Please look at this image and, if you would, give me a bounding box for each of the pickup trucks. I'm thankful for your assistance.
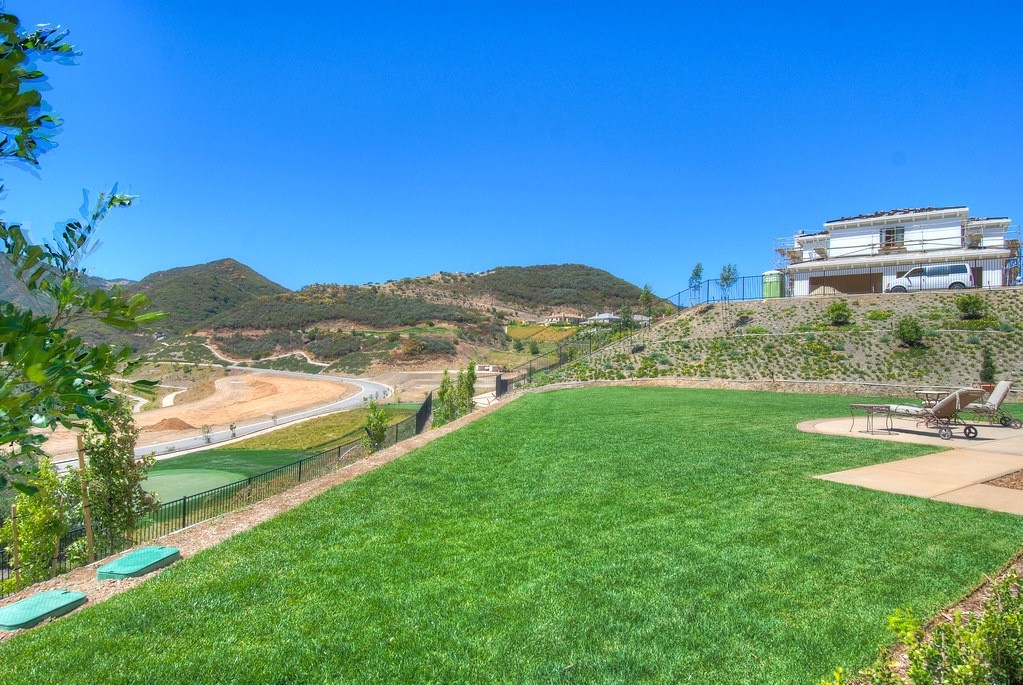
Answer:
[884,262,977,291]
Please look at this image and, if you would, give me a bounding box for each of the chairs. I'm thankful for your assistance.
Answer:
[885,389,985,437]
[923,381,1022,427]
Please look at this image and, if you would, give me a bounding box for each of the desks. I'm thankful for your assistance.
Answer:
[849,404,893,436]
[915,391,950,429]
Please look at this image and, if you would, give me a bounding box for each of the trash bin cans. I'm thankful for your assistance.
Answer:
[761,269,783,299]
